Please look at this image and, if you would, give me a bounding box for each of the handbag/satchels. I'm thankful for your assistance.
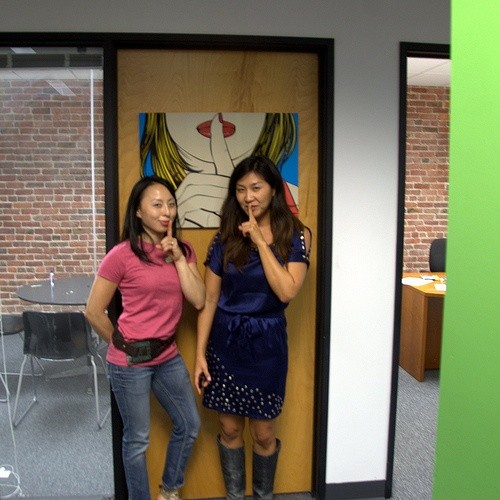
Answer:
[111,324,176,366]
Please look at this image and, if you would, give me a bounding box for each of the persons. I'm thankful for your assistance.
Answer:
[84,176,207,500]
[193,156,313,500]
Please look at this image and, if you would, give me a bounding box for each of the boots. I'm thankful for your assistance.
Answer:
[252,438,281,500]
[216,434,246,500]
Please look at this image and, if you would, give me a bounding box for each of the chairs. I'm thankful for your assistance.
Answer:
[0,314,45,402]
[12,311,111,430]
[430,237,447,271]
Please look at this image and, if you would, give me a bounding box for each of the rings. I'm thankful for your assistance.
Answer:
[171,241,174,246]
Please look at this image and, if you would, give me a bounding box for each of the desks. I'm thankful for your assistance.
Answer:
[17,274,107,392]
[402,271,447,381]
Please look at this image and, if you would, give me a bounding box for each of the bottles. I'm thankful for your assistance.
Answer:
[49,270,55,286]
